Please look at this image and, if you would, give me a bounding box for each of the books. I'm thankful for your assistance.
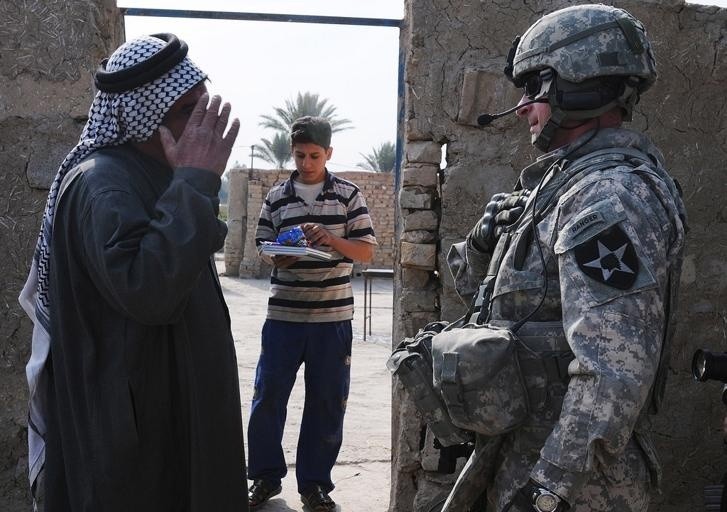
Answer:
[262,245,332,262]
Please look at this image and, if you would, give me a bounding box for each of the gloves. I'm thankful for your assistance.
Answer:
[472,190,531,251]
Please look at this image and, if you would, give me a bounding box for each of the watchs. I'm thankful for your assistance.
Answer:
[521,481,570,512]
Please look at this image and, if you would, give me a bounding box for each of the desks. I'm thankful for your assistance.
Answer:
[361,268,395,342]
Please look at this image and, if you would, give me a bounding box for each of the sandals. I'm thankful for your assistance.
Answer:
[246,478,283,508]
[299,484,335,511]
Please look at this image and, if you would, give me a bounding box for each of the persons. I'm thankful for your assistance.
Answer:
[17,34,249,512]
[247,116,379,512]
[385,3,689,512]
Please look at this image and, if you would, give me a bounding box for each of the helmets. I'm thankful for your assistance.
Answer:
[507,2,658,89]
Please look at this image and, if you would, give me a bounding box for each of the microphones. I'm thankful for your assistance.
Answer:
[477,93,548,126]
